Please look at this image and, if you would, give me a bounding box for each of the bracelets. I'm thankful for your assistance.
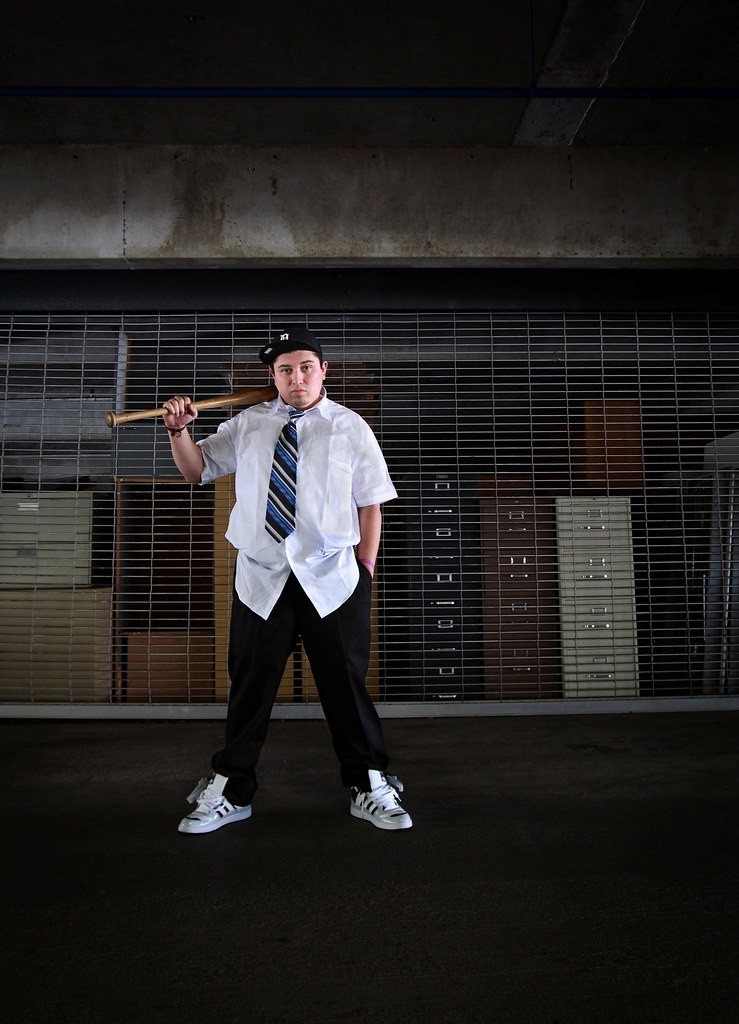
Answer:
[359,559,375,568]
[166,424,187,437]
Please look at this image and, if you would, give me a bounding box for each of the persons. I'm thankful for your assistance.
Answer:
[162,329,413,833]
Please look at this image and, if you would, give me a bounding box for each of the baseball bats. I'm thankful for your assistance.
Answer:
[105,385,278,428]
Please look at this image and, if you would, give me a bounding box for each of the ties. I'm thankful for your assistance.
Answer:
[264,409,306,544]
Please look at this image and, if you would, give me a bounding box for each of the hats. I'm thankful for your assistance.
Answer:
[259,329,323,364]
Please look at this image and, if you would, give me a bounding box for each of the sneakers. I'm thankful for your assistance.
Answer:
[178,773,252,834]
[349,767,412,829]
